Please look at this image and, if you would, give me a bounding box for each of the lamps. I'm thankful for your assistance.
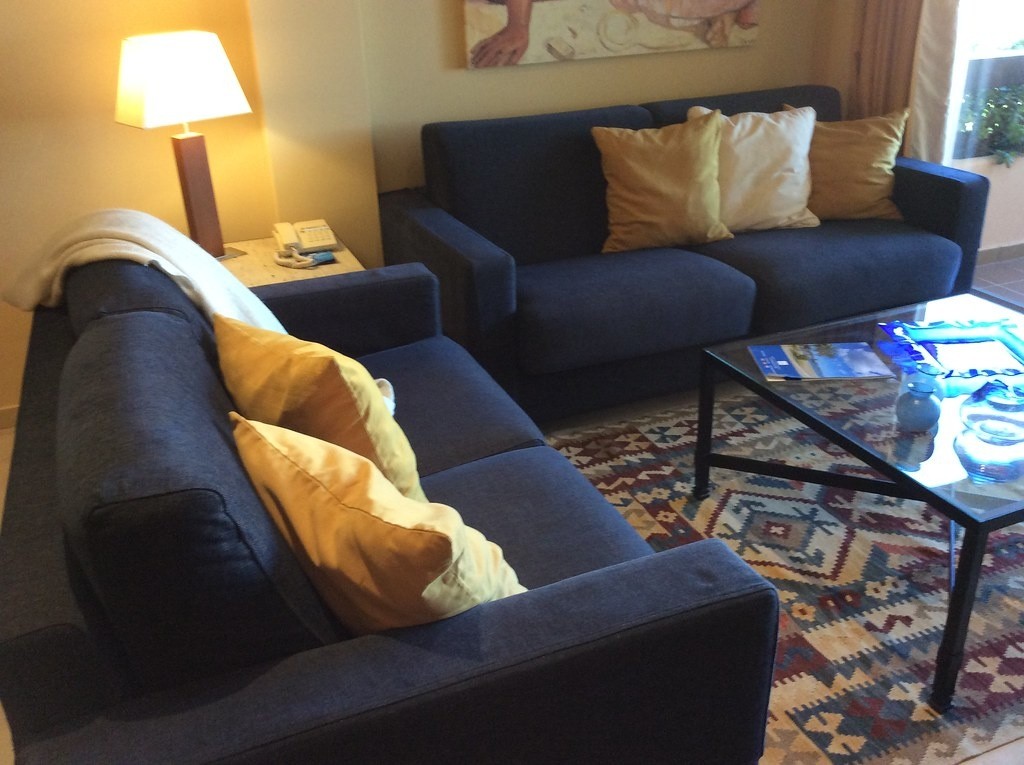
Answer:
[114,31,253,258]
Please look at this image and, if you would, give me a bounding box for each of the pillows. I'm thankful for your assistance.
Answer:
[222,414,529,637]
[210,313,430,518]
[672,96,821,235]
[780,102,912,226]
[591,106,736,255]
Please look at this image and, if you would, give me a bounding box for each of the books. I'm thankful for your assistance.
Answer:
[747,342,897,382]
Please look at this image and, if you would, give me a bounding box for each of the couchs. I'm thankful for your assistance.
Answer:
[1,261,780,765]
[378,85,991,424]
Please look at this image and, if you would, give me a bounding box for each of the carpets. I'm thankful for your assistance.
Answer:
[542,377,1023,763]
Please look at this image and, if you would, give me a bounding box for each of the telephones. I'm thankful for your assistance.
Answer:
[271,219,336,256]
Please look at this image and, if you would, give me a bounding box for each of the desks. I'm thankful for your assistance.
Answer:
[695,292,1024,715]
[216,231,366,287]
